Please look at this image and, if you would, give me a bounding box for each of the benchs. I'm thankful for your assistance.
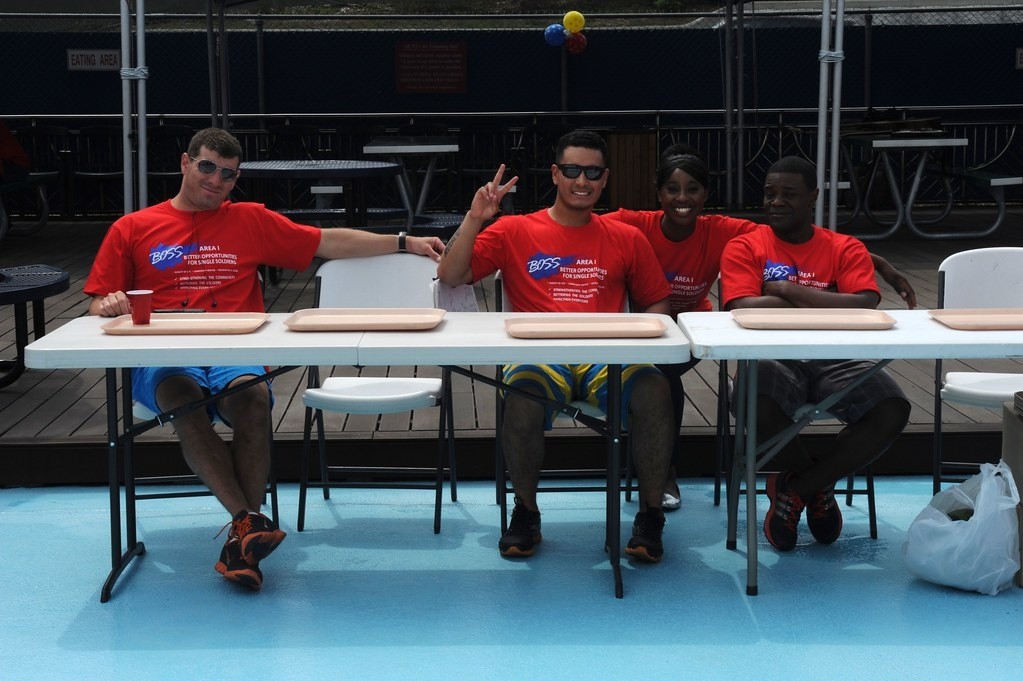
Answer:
[348,205,469,246]
[0,265,69,388]
[926,165,1023,239]
[824,176,850,227]
[0,171,59,241]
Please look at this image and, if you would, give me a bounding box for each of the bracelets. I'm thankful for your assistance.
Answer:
[398,231,409,253]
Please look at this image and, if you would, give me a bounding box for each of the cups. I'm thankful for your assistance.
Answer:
[126,290,153,325]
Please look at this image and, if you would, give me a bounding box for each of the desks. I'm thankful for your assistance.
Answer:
[23,312,691,604]
[237,134,459,286]
[839,135,968,240]
[677,308,1023,596]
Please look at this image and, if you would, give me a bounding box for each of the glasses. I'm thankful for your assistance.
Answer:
[190,156,237,182]
[556,164,605,180]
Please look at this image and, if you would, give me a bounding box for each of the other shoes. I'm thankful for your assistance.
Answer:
[662,484,681,508]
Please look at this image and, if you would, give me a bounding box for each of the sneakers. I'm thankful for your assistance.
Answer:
[499,496,542,556]
[213,512,287,567]
[764,473,805,551]
[806,479,842,543]
[214,537,263,590]
[625,512,666,561]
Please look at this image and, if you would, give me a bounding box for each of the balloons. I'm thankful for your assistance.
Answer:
[563,11,585,33]
[544,24,566,47]
[565,33,587,55]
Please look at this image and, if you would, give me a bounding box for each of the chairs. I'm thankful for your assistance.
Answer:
[494,267,879,550]
[933,247,1023,495]
[134,252,459,536]
[15,125,614,220]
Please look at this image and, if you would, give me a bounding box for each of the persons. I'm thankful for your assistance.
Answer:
[436,132,676,564]
[718,156,912,551]
[320,381,323,386]
[81,127,444,592]
[598,144,919,509]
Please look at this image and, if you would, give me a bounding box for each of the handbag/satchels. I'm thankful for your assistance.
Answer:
[904,458,1021,595]
